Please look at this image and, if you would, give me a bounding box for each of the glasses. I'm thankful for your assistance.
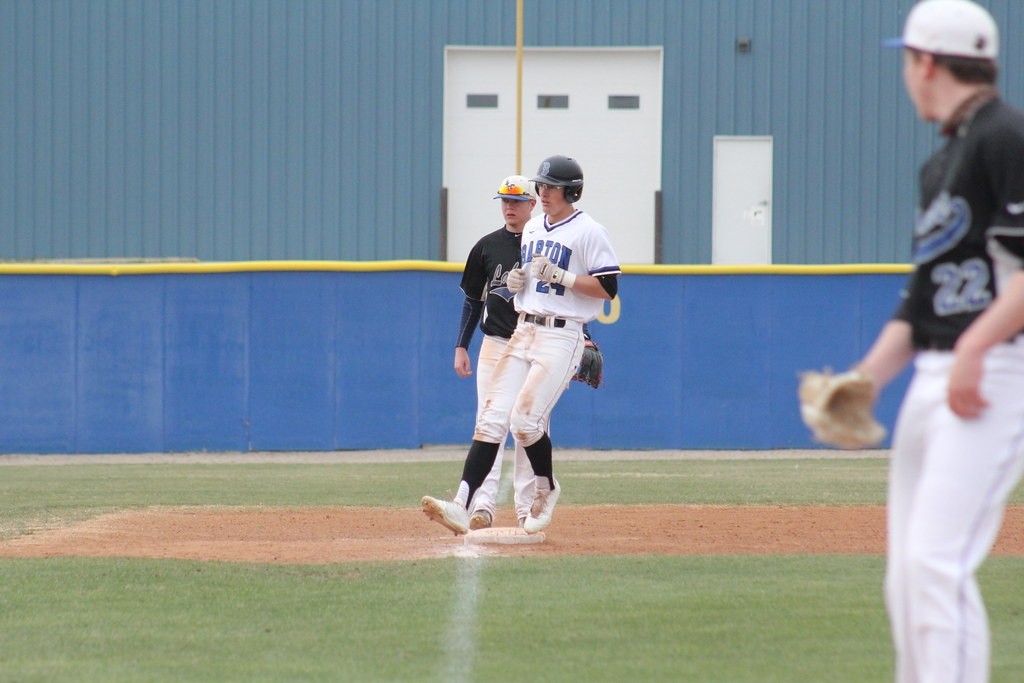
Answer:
[499,185,530,195]
[537,182,565,190]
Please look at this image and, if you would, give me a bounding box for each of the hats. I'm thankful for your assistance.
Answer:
[882,1,999,57]
[494,174,537,202]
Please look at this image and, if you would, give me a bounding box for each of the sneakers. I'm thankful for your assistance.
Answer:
[523,476,561,533]
[422,495,471,534]
[519,517,527,527]
[469,512,491,530]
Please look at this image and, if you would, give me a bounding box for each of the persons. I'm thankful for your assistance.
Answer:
[797,1,1024,683]
[421,155,622,535]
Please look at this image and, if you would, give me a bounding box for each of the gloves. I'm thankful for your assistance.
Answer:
[532,253,576,289]
[507,268,527,292]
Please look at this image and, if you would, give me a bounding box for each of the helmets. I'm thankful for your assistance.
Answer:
[527,155,583,202]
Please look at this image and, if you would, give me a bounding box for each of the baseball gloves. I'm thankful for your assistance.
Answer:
[797,370,890,453]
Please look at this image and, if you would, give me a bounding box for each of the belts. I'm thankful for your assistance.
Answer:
[524,313,566,328]
